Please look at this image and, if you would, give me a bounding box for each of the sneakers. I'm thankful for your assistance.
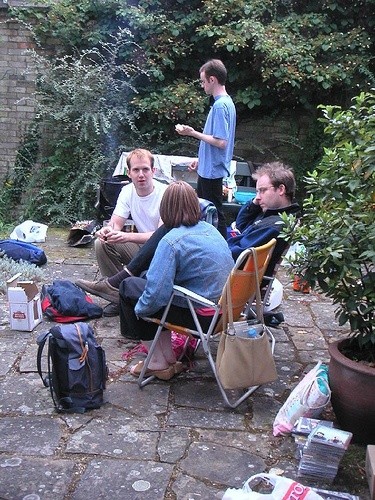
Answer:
[75,277,122,305]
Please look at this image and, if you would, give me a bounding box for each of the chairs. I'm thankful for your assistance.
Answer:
[94,180,300,409]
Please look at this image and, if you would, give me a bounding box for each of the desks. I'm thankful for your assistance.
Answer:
[153,153,252,188]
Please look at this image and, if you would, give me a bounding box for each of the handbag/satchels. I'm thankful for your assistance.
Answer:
[273,361,331,436]
[10,220,49,243]
[67,220,99,247]
[0,240,47,265]
[41,279,102,323]
[222,472,324,500]
[215,247,279,390]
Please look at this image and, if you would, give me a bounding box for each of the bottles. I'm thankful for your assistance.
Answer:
[226,318,266,338]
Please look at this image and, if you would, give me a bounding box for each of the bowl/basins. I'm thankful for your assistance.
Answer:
[234,192,257,205]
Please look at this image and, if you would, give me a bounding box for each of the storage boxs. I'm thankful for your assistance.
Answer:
[67,220,99,247]
[7,273,43,332]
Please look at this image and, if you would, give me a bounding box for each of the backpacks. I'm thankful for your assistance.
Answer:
[36,322,109,413]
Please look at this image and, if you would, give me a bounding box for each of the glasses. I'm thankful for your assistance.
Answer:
[256,186,275,194]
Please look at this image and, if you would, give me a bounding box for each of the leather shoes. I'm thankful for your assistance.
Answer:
[102,301,119,317]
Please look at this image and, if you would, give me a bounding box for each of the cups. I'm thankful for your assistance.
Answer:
[103,220,114,243]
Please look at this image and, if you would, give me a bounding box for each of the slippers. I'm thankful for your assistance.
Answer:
[129,362,182,380]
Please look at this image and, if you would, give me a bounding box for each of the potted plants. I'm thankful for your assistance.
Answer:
[274,93,375,445]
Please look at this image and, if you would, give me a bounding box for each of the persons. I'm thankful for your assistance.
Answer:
[117,181,238,381]
[95,149,171,318]
[175,59,237,242]
[76,162,300,306]
[313,431,345,445]
